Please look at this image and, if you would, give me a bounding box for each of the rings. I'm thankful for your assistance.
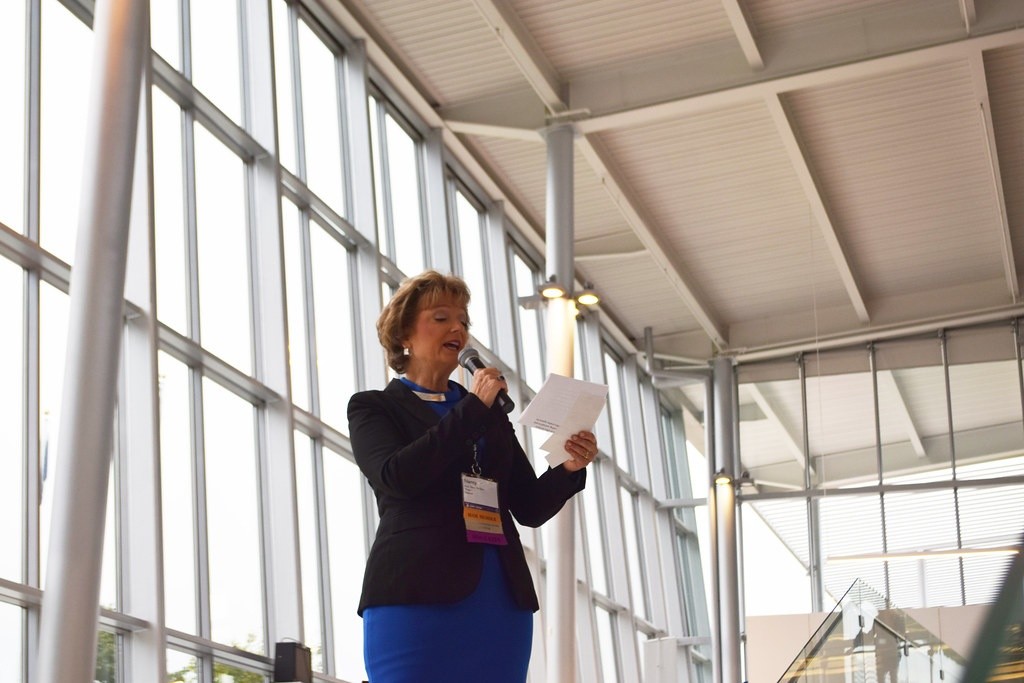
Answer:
[497,376,505,381]
[584,452,589,459]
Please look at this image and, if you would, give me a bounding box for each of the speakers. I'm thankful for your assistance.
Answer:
[274,642,312,683]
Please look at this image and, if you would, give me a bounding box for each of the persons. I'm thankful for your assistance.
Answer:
[346,269,598,683]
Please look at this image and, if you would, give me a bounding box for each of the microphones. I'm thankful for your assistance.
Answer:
[457,348,515,413]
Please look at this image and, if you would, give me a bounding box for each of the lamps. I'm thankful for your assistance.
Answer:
[536,281,568,299]
[574,288,602,306]
